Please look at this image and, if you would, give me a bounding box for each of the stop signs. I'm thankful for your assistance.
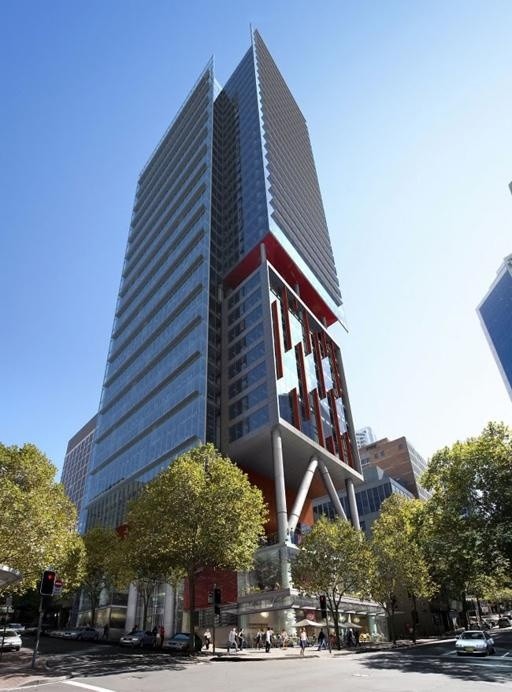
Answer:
[54,579,62,595]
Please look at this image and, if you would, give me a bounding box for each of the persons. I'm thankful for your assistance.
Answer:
[346,628,353,648]
[299,626,309,656]
[102,623,110,640]
[329,633,337,648]
[312,632,315,639]
[270,627,275,633]
[353,627,362,647]
[265,626,272,652]
[281,628,288,648]
[238,627,249,651]
[203,627,212,650]
[317,627,327,650]
[254,628,260,650]
[159,625,165,648]
[260,628,266,640]
[152,625,158,647]
[226,627,240,652]
[131,624,138,631]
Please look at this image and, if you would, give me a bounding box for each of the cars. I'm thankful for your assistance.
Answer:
[120,630,159,648]
[0,623,100,651]
[162,632,202,656]
[456,630,495,655]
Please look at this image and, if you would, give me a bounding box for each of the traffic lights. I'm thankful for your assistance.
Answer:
[215,588,221,604]
[40,570,56,597]
[320,595,327,610]
